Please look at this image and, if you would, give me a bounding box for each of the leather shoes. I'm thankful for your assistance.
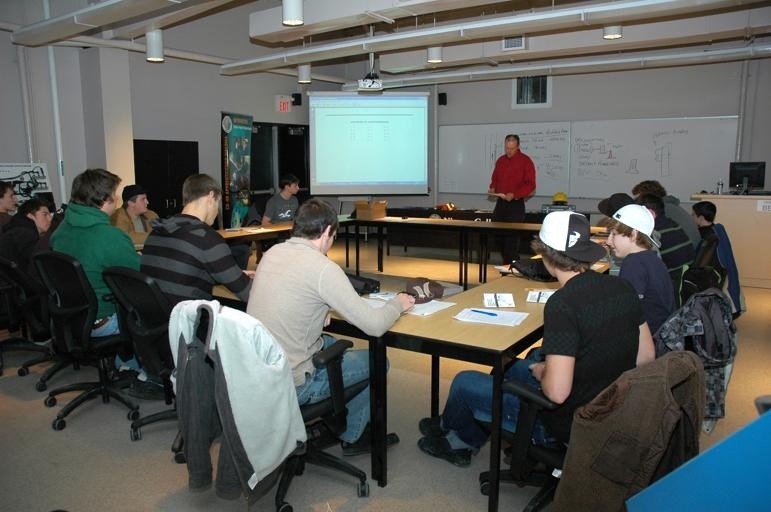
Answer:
[342,423,399,456]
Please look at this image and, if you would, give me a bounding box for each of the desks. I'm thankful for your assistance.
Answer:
[375,257,611,510]
[353,207,581,291]
[212,270,375,480]
[135,211,363,279]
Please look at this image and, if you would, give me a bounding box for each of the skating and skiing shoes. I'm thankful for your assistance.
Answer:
[420,416,446,436]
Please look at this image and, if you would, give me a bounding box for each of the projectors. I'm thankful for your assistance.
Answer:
[341,78,385,93]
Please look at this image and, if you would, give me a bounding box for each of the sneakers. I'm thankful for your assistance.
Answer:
[419,436,472,465]
[129,373,166,400]
[112,365,140,386]
[34,332,56,345]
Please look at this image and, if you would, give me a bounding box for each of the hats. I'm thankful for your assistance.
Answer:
[406,278,444,304]
[123,185,151,201]
[599,194,636,218]
[613,204,660,248]
[539,211,607,262]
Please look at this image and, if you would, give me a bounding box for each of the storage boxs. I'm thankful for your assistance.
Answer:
[354,198,390,226]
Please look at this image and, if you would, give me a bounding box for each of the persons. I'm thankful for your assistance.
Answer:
[631,191,698,313]
[0,181,18,230]
[48,167,169,401]
[416,208,658,467]
[594,192,636,221]
[525,204,678,363]
[139,173,251,317]
[260,173,301,228]
[0,197,64,346]
[110,182,162,247]
[689,201,721,277]
[631,177,704,262]
[486,132,538,223]
[245,195,417,458]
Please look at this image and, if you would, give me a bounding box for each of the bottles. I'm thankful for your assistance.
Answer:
[717,179,724,195]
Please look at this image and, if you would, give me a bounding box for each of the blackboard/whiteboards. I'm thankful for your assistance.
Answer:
[438,119,570,200]
[569,114,738,204]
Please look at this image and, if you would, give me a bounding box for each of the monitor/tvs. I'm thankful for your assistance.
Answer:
[728,160,766,196]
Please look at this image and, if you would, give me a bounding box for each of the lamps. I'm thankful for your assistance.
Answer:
[8,0,196,49]
[342,45,771,95]
[218,1,756,79]
[601,24,623,42]
[427,46,443,66]
[297,63,311,85]
[143,23,165,64]
[281,0,305,29]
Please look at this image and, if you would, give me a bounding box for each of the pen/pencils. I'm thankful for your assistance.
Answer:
[537,292,541,303]
[471,310,497,316]
[494,293,499,307]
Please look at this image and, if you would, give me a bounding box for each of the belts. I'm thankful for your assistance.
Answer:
[94,316,109,329]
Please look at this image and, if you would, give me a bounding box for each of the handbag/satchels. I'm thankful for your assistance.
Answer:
[346,273,380,293]
[510,254,559,281]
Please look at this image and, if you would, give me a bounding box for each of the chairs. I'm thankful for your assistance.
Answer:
[33,249,144,432]
[1,245,98,391]
[478,347,706,512]
[654,288,738,430]
[169,300,370,512]
[101,267,184,464]
[682,235,720,296]
